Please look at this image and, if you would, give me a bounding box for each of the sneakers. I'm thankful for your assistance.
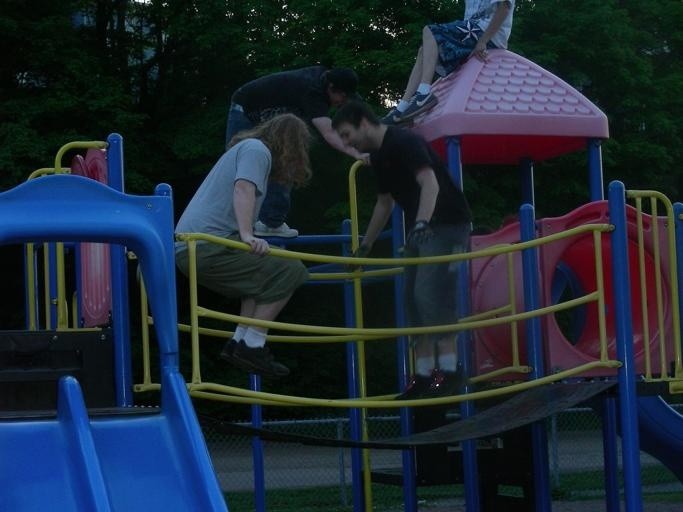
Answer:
[380,90,439,128]
[253,219,300,238]
[221,339,290,381]
[394,369,459,401]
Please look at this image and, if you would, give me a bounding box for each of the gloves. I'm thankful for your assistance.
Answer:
[407,219,437,249]
[343,246,370,282]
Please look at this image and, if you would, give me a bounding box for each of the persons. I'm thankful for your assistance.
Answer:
[223,64,372,241]
[172,111,313,380]
[376,0,515,124]
[330,99,476,400]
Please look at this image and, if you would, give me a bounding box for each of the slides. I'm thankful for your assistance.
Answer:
[0,370,228,512]
